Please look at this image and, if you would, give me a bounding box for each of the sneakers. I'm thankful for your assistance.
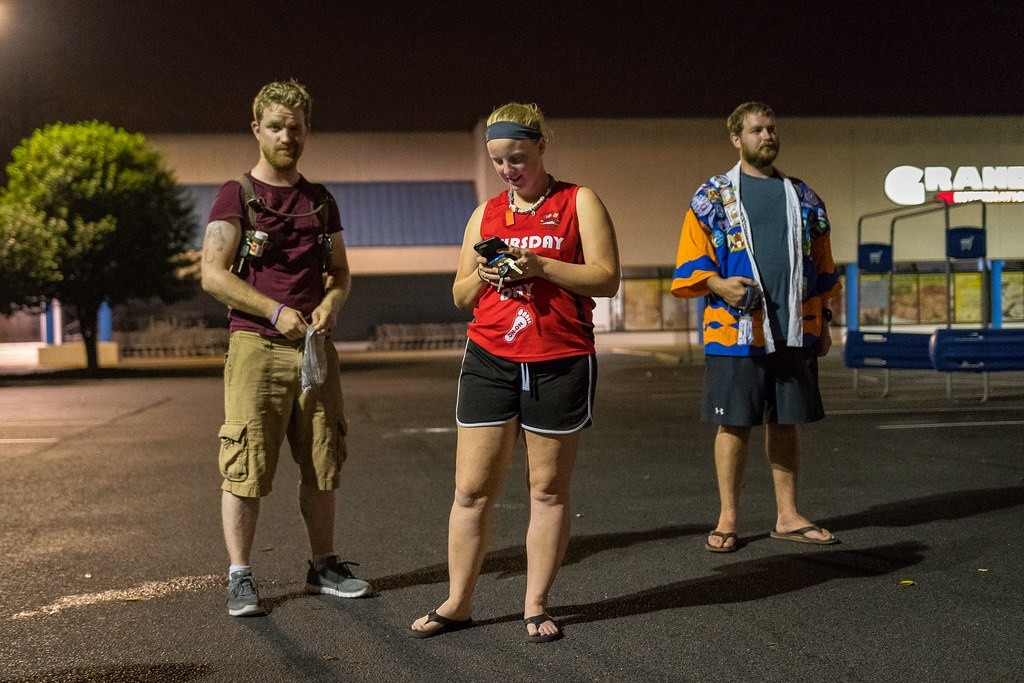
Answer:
[224,569,265,617]
[304,555,373,597]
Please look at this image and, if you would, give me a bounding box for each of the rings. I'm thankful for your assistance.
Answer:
[488,279,491,284]
[328,328,332,335]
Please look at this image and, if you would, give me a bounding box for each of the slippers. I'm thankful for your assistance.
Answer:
[704,530,737,552]
[406,609,472,639]
[770,525,839,544]
[523,614,557,643]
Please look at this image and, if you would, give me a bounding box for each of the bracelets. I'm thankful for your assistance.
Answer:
[477,268,486,282]
[271,304,285,325]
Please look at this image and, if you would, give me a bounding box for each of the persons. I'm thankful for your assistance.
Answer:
[671,102,840,552]
[408,102,620,641]
[201,79,371,617]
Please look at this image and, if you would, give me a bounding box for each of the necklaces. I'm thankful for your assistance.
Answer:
[508,174,555,213]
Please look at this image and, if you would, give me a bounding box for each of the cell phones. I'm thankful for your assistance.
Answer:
[473,236,508,262]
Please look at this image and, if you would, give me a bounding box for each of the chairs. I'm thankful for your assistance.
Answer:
[60,315,228,356]
[375,322,468,351]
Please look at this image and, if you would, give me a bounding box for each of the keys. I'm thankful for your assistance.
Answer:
[497,258,523,292]
